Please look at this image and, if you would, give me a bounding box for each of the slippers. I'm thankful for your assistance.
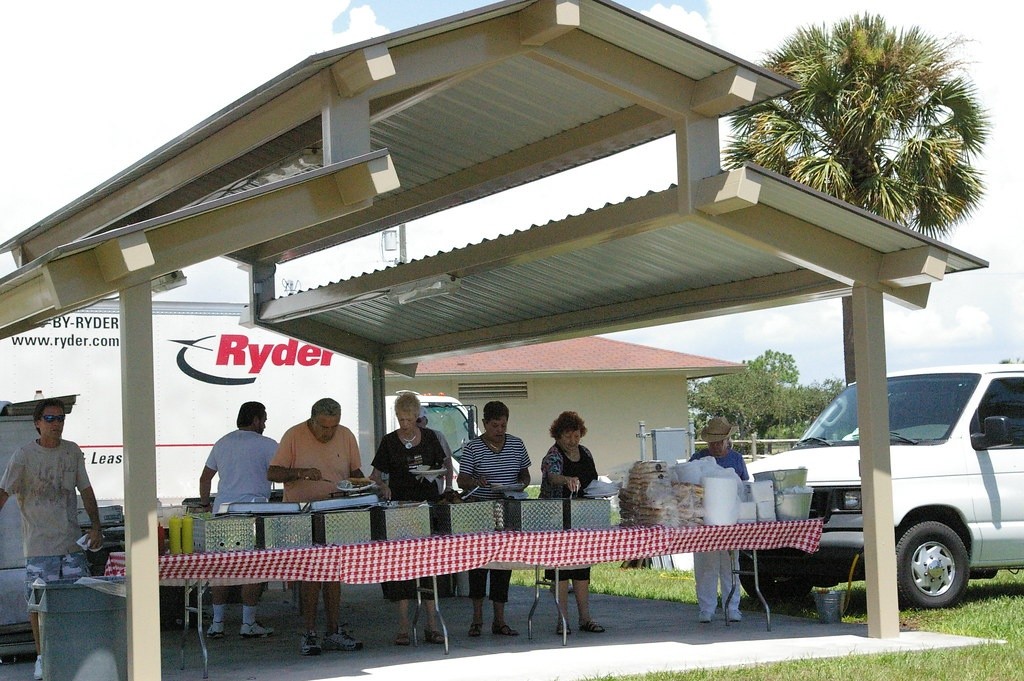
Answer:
[557,618,571,635]
[579,620,605,633]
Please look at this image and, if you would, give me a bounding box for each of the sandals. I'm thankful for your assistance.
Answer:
[396,632,411,645]
[424,629,445,643]
[491,622,519,636]
[468,622,483,636]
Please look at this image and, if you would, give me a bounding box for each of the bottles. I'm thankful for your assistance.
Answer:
[181,518,194,553]
[34,390,44,400]
[169,518,182,554]
[156,498,163,518]
[158,522,165,556]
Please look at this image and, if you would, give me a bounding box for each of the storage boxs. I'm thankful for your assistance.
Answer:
[564,498,610,529]
[313,512,374,546]
[504,499,564,531]
[192,518,254,553]
[433,501,497,534]
[370,506,431,541]
[257,515,315,548]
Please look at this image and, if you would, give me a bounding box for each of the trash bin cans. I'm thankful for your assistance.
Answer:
[28,572,129,680]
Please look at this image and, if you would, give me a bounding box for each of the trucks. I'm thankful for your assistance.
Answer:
[0,297,484,535]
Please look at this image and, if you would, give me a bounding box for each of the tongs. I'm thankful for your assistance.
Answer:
[305,477,333,483]
[459,484,480,500]
[570,480,580,499]
[385,499,392,506]
[297,501,310,513]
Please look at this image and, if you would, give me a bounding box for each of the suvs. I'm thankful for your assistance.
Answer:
[737,363,1023,614]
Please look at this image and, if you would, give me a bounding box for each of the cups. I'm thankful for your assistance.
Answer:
[417,466,430,471]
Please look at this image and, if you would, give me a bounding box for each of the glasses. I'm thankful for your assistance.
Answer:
[416,418,423,424]
[44,415,66,423]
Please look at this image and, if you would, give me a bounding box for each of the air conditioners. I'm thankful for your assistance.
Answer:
[151,270,186,292]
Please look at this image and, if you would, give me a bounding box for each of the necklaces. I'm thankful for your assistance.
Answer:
[401,431,418,448]
[485,437,505,450]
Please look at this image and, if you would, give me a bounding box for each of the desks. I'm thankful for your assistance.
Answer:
[103,517,824,679]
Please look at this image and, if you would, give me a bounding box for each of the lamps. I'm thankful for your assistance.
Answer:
[298,148,328,167]
[388,274,460,305]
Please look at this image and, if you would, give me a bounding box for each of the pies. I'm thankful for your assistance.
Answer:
[618,460,703,526]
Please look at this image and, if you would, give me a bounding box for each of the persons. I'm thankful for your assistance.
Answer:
[195,402,278,637]
[370,393,454,645]
[457,401,532,635]
[688,417,749,622]
[538,411,606,634]
[267,398,365,655]
[0,398,103,679]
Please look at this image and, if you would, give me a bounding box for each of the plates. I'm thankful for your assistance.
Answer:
[584,493,617,497]
[395,502,421,507]
[409,469,447,473]
[337,480,376,491]
[490,483,525,489]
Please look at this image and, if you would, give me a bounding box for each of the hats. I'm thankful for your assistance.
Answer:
[701,417,738,442]
[418,406,427,418]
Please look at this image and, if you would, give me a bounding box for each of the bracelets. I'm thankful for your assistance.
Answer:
[200,503,210,507]
[92,528,102,533]
[297,469,303,479]
[446,486,453,488]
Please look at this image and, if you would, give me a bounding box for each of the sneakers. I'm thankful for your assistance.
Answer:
[34,659,42,679]
[240,621,274,636]
[207,621,224,639]
[321,627,363,651]
[302,634,322,655]
[698,611,713,622]
[727,610,742,621]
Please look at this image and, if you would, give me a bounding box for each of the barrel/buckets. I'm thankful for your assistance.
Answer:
[752,469,808,493]
[775,492,814,520]
[815,591,846,623]
